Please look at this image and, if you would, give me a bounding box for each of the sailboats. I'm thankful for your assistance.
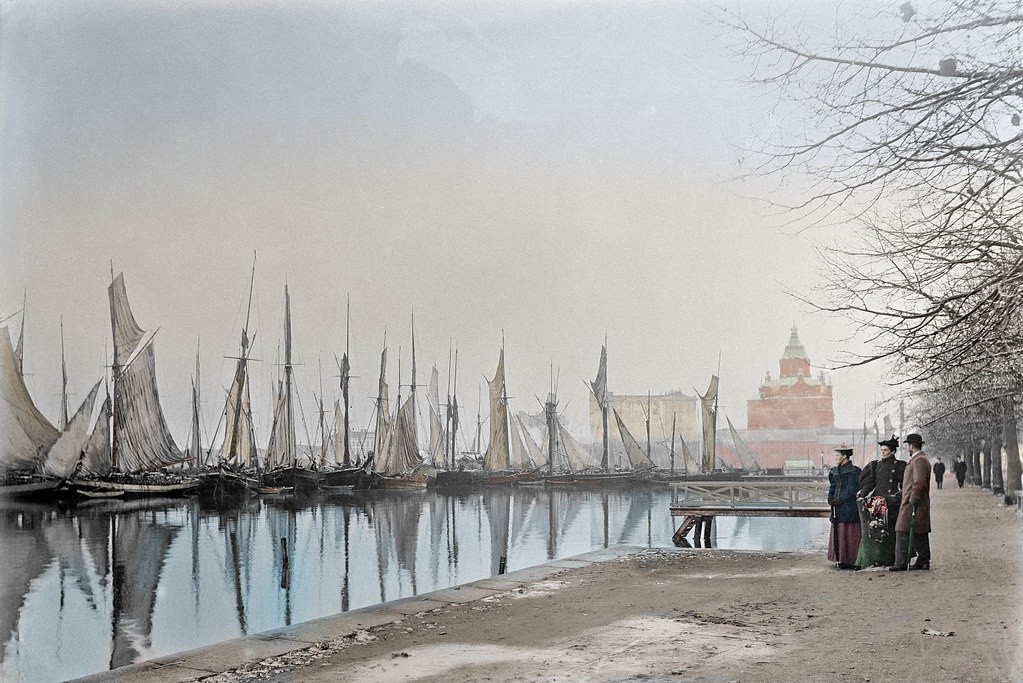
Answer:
[0,251,764,508]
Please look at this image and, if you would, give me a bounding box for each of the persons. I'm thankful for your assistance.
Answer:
[954,455,967,487]
[932,457,946,489]
[860,437,910,569]
[824,443,867,569]
[888,433,933,572]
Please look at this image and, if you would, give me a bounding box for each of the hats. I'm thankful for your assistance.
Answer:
[903,434,926,444]
[832,441,854,451]
[877,434,901,448]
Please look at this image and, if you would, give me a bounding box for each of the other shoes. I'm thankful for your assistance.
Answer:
[889,565,908,571]
[909,560,930,570]
[836,563,852,569]
[854,566,862,571]
